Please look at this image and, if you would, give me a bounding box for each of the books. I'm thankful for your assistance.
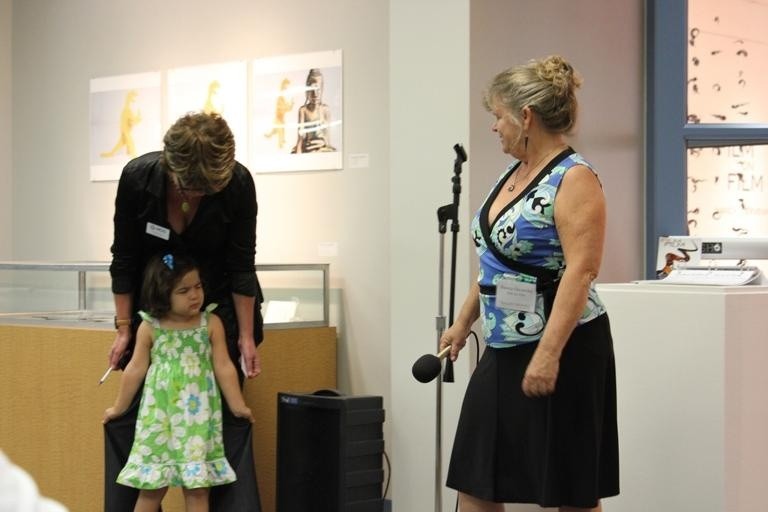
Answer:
[633,265,763,286]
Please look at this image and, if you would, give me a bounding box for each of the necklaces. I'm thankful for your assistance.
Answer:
[179,192,193,214]
[507,143,560,193]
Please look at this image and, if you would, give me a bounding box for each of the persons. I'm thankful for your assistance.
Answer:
[101,247,256,512]
[440,52,622,512]
[105,110,265,412]
[290,67,337,152]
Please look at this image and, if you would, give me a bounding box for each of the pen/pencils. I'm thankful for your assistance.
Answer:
[98,354,124,385]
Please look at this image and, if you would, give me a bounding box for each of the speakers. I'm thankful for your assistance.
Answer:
[274,388,392,511]
[105,419,262,511]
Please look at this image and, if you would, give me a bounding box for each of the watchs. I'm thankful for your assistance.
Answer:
[114,315,134,330]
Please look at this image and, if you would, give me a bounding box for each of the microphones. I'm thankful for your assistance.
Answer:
[411,344,452,383]
